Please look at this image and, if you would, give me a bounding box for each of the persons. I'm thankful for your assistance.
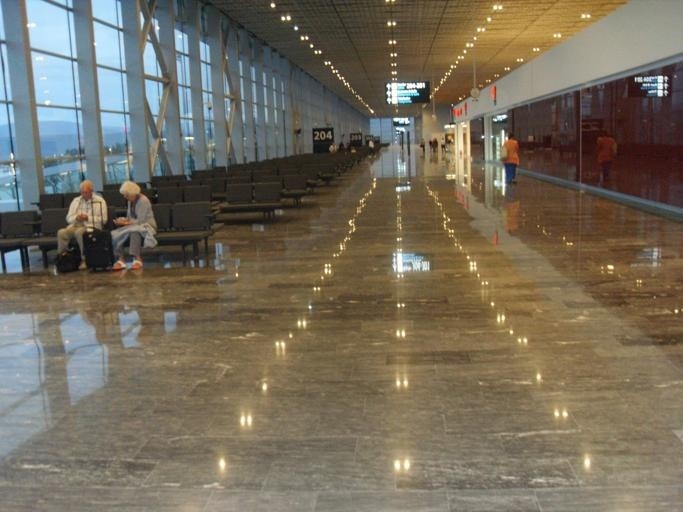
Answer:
[338,141,344,149]
[56,177,108,270]
[109,180,157,270]
[501,132,520,184]
[596,130,616,178]
[432,138,437,152]
[421,138,426,152]
[429,140,432,150]
[440,137,446,152]
[328,143,336,152]
[365,137,376,160]
[435,137,439,147]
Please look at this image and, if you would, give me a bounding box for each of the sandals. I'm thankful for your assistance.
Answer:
[113,260,144,270]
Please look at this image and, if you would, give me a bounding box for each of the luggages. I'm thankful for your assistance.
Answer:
[83,201,113,271]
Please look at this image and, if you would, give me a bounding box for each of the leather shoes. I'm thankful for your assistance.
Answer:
[78,261,87,269]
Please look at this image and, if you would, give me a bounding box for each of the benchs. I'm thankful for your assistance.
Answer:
[1,200,213,271]
[35,182,157,208]
[151,154,360,221]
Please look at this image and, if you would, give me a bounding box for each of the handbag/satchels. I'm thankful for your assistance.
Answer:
[499,147,507,160]
[54,245,80,273]
